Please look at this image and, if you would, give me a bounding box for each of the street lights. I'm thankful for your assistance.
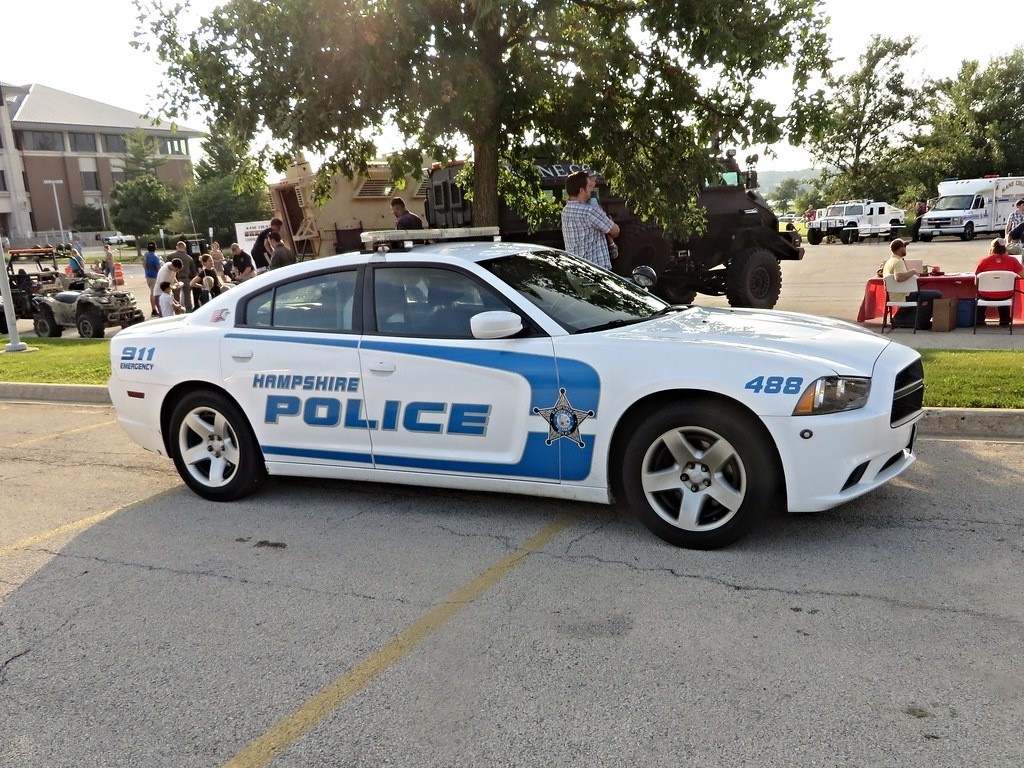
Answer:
[43,179,65,244]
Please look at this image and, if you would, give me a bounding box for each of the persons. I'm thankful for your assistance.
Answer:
[209,241,230,284]
[1005,199,1024,237]
[561,171,620,271]
[785,202,924,242]
[267,231,297,270]
[190,254,222,299]
[251,217,283,276]
[974,238,1024,329]
[1007,221,1024,248]
[882,238,942,330]
[1,234,208,317]
[391,197,425,244]
[231,242,257,281]
[198,276,216,307]
[582,169,619,259]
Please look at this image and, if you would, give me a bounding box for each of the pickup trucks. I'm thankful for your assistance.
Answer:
[102,231,135,246]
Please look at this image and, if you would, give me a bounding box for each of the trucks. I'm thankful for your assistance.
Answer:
[424,149,806,312]
[917,175,1024,242]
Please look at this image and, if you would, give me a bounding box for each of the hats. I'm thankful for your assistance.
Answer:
[891,239,909,250]
[585,168,597,178]
[991,238,1006,247]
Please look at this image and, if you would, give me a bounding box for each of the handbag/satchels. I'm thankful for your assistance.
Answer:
[1005,239,1024,254]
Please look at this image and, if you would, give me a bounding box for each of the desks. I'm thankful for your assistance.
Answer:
[843,226,907,245]
[857,273,1024,322]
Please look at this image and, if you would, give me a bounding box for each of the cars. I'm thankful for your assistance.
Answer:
[777,210,809,223]
[105,227,929,552]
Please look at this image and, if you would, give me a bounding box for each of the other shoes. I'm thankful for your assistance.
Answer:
[973,320,986,326]
[916,321,932,330]
[999,319,1012,325]
[892,316,915,328]
[609,243,619,260]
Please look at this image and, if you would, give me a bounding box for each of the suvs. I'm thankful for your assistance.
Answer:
[803,199,905,245]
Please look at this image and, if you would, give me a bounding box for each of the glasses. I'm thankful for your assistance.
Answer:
[901,245,906,248]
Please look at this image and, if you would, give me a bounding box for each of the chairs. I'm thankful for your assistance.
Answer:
[973,270,1017,335]
[882,274,921,334]
[905,260,924,273]
[877,223,891,246]
[427,278,471,329]
[37,267,57,282]
[16,269,34,289]
[857,224,872,245]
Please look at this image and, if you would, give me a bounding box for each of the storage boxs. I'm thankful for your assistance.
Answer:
[932,298,958,332]
[957,300,975,328]
